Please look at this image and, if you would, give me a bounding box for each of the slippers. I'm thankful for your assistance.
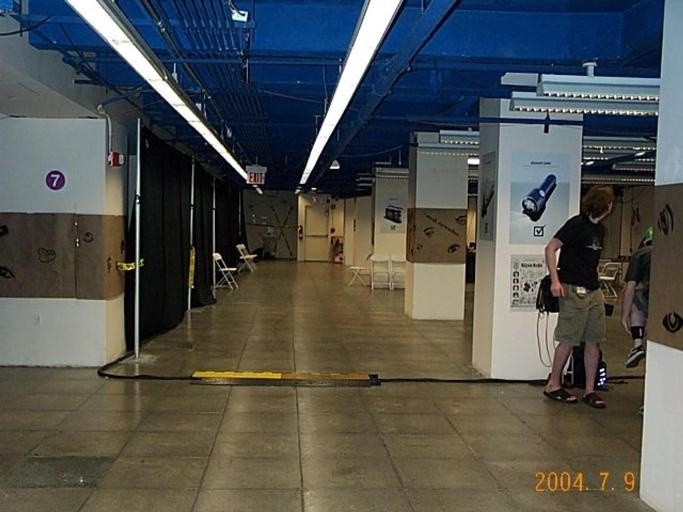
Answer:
[543,388,577,404]
[581,393,606,408]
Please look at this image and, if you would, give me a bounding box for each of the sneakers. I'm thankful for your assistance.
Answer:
[624,344,645,367]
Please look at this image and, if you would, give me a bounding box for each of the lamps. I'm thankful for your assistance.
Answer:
[330,160,340,169]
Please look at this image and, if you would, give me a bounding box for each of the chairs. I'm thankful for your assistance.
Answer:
[348,253,406,291]
[597,263,621,298]
[213,252,238,290]
[236,243,258,273]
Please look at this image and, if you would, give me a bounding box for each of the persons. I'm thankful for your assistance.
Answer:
[545,184,614,409]
[619,226,653,418]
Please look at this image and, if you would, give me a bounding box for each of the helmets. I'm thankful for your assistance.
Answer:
[643,227,653,246]
